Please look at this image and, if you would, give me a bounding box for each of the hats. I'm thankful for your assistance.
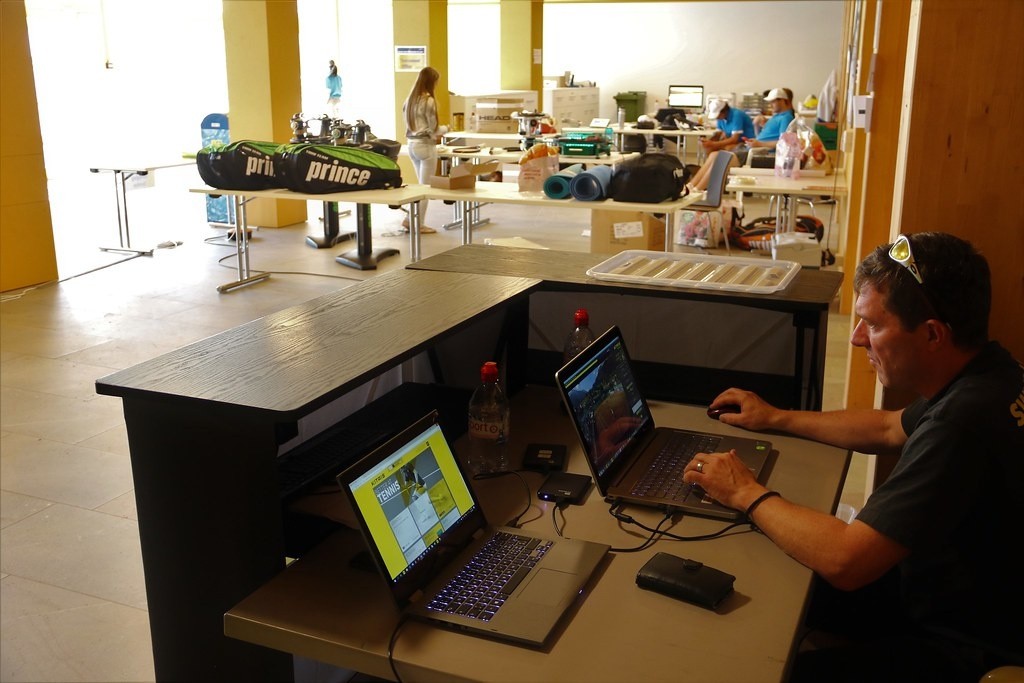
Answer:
[763,88,786,101]
[708,99,726,119]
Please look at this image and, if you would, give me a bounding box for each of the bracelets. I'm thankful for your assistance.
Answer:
[743,489,780,525]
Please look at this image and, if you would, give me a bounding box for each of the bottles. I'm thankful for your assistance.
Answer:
[466,362,510,473]
[564,307,595,365]
[618,107,626,129]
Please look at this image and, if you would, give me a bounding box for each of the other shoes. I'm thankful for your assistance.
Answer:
[417,226,436,235]
[403,218,409,229]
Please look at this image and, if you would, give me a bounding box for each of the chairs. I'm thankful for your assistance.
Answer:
[745,147,776,169]
[681,149,734,257]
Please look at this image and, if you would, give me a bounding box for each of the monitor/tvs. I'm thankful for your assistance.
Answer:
[667,84,704,108]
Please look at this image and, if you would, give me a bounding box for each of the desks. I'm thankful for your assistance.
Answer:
[89,116,848,294]
[225,385,854,683]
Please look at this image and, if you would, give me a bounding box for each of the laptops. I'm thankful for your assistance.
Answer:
[555,323,772,520]
[336,408,612,645]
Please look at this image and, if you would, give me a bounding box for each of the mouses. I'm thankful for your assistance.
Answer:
[708,404,740,419]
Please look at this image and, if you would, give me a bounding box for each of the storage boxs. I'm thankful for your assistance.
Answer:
[590,209,673,255]
[476,98,523,134]
[430,159,498,190]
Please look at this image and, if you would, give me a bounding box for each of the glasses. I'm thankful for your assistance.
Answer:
[888,235,922,285]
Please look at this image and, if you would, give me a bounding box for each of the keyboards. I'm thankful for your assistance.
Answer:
[277,428,392,505]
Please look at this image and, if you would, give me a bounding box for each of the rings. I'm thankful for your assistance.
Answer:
[697,462,704,472]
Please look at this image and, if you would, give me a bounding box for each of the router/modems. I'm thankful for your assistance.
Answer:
[538,473,592,503]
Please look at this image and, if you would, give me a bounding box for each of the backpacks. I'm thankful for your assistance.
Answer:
[738,215,824,253]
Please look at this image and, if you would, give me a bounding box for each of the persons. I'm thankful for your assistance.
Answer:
[400,465,427,501]
[753,88,794,126]
[683,233,1024,683]
[681,87,795,191]
[700,99,755,156]
[402,67,449,233]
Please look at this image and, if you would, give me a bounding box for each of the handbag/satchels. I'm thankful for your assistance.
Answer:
[776,117,834,178]
[611,153,690,203]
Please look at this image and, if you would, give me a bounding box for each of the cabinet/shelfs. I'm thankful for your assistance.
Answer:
[542,87,600,132]
